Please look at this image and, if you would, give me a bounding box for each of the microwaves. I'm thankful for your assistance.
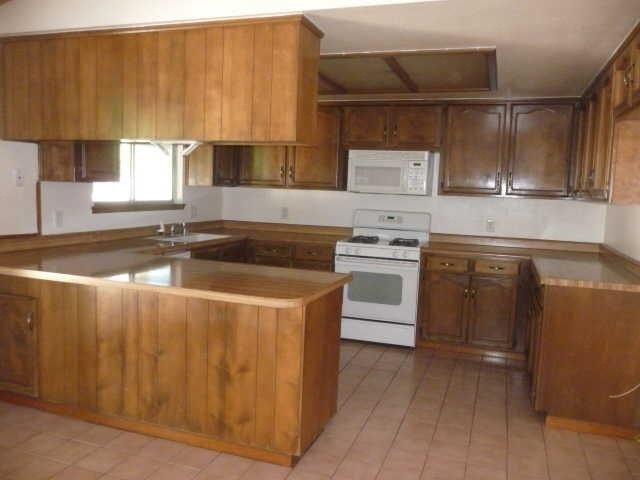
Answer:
[347,148,440,197]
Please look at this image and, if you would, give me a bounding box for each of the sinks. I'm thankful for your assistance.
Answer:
[147,231,232,245]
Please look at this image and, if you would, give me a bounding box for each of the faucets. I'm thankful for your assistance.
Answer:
[155,222,189,235]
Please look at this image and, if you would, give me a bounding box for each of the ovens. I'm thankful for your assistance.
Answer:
[333,255,420,348]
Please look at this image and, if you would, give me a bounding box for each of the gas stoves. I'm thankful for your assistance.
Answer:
[335,234,427,261]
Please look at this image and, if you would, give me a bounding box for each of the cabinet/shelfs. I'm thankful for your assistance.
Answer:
[523,253,640,440]
[247,238,335,275]
[439,103,574,200]
[38,141,120,182]
[212,101,344,191]
[420,251,523,360]
[614,21,640,117]
[342,105,442,149]
[192,236,245,264]
[570,52,639,203]
